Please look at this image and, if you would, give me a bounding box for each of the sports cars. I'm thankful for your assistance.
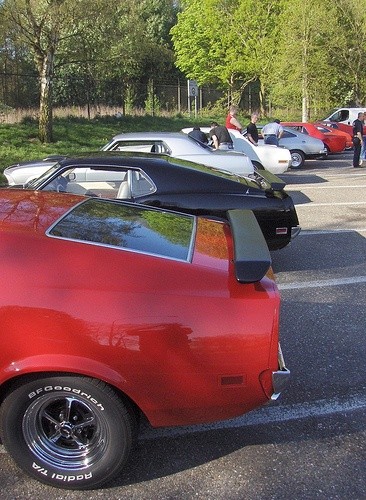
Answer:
[114,120,366,175]
[0,153,302,251]
[0,188,293,490]
[2,130,256,189]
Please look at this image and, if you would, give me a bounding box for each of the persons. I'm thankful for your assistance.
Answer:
[209,122,233,150]
[261,119,284,146]
[226,105,242,132]
[188,126,208,144]
[352,112,366,168]
[247,112,259,145]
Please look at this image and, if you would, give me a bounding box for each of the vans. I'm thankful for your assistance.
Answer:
[318,107,366,128]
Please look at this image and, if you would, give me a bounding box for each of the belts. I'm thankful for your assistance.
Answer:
[353,135,358,137]
[219,142,233,145]
[264,134,276,136]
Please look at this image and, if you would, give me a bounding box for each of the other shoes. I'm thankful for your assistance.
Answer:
[354,165,363,168]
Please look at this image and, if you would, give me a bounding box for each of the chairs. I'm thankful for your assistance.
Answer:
[117,180,128,198]
